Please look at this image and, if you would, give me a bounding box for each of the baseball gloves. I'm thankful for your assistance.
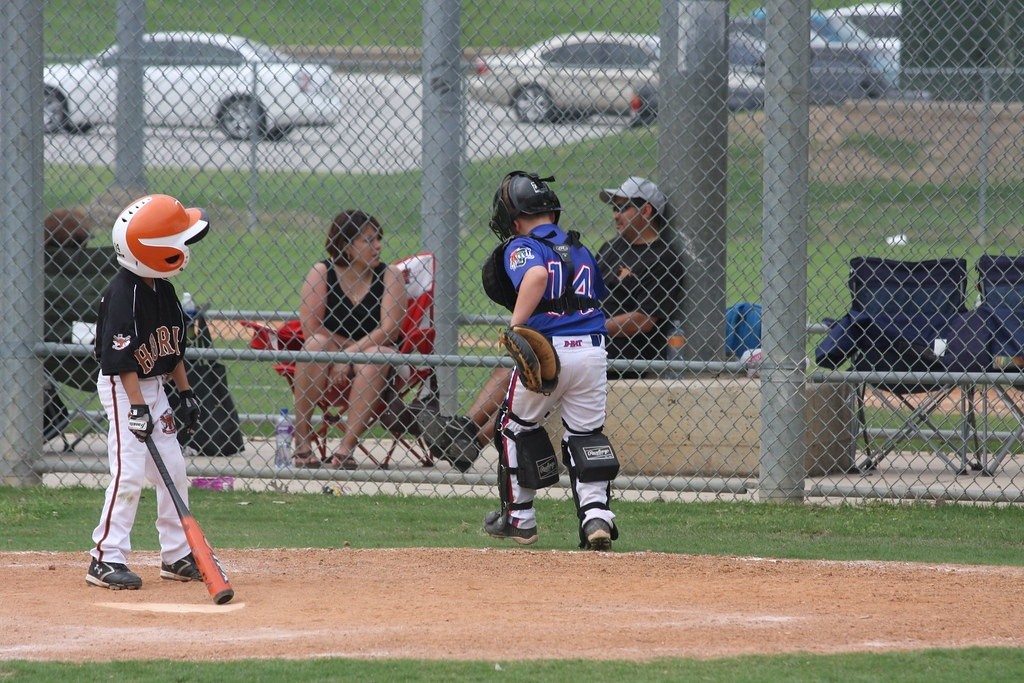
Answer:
[504,324,561,397]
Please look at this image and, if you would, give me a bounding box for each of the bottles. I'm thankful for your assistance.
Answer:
[180,292,197,347]
[664,319,686,380]
[740,349,811,378]
[274,409,293,468]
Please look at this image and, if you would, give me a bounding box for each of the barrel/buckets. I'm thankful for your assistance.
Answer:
[192,474,233,492]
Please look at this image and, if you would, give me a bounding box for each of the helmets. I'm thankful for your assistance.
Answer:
[112,193,212,279]
[486,170,565,236]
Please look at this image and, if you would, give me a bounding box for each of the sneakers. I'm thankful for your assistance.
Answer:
[414,412,482,474]
[160,551,204,582]
[584,519,613,551]
[483,512,538,545]
[86,557,143,590]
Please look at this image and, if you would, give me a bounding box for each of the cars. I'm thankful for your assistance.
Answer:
[41,30,341,141]
[467,25,765,123]
[707,3,932,99]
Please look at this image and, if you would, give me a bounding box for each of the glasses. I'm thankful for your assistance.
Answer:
[612,202,640,213]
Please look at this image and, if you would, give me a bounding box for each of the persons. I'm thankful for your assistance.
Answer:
[85,193,210,591]
[292,211,408,470]
[416,176,685,472]
[484,171,620,551]
[44,209,96,444]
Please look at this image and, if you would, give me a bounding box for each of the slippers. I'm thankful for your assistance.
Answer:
[330,452,358,471]
[292,450,321,468]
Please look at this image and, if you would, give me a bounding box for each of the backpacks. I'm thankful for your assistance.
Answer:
[725,302,764,357]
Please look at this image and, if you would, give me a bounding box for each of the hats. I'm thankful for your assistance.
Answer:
[599,176,666,221]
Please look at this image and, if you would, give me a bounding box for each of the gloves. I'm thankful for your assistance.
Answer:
[128,403,154,443]
[178,389,199,429]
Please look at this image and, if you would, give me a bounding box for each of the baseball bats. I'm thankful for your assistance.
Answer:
[145,431,234,607]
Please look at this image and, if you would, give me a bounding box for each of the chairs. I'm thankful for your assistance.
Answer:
[43,246,212,453]
[942,253,1024,478]
[814,257,983,476]
[247,250,440,469]
